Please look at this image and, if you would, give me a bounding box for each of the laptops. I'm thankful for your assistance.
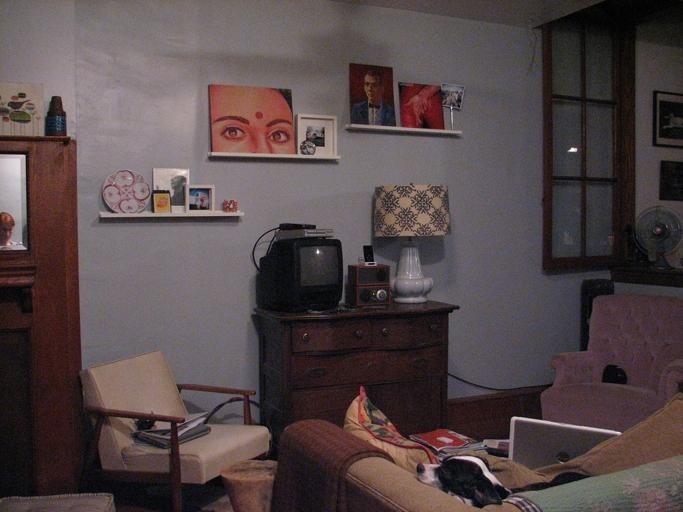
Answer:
[509,416,621,474]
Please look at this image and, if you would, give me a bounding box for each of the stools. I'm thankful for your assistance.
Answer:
[0,492,117,512]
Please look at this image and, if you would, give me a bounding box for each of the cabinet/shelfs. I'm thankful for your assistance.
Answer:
[98,124,461,218]
[251,300,461,462]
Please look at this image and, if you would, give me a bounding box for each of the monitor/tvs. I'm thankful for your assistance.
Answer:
[256,238,343,313]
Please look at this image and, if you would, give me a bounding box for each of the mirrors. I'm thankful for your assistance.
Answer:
[1,139,39,268]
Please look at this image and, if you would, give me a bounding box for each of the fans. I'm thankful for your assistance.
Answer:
[632,206,683,272]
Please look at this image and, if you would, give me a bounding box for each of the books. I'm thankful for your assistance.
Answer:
[129,409,213,450]
[407,427,488,464]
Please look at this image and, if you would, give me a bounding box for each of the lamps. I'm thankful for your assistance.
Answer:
[372,183,450,305]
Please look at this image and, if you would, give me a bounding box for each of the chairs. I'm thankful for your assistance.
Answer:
[540,295,683,434]
[77,348,270,512]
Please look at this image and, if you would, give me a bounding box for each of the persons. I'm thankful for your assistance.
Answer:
[407,83,441,127]
[208,84,295,155]
[169,175,186,206]
[0,211,22,247]
[348,67,397,125]
[442,88,463,109]
[305,126,315,141]
[198,193,209,210]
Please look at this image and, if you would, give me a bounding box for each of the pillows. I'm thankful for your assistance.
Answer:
[339,384,440,480]
[534,392,683,482]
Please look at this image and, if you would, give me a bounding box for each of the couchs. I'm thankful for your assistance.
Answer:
[276,420,683,512]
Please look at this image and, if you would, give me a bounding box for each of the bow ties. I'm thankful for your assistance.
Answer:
[368,102,382,110]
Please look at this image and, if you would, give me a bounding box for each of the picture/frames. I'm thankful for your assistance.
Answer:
[153,168,190,214]
[294,113,338,158]
[653,90,683,148]
[659,160,683,201]
[185,185,215,216]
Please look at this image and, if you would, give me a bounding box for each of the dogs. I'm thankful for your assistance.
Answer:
[415,452,589,510]
